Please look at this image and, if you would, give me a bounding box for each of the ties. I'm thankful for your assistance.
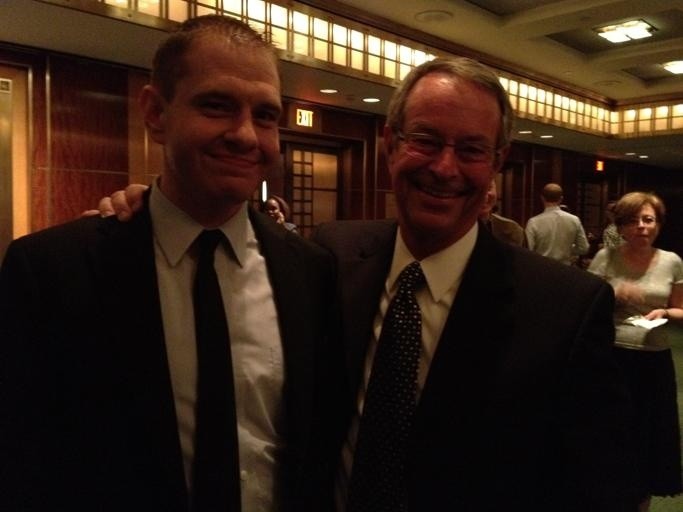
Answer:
[187,229,244,512]
[353,263,427,510]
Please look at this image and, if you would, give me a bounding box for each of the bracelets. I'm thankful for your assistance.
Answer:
[664,309,669,316]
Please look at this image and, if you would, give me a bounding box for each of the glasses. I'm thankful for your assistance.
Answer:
[394,124,499,163]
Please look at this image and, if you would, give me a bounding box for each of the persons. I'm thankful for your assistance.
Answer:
[603,202,625,246]
[480,179,528,255]
[0,17,338,511]
[589,193,683,511]
[525,184,590,266]
[259,195,298,234]
[83,56,640,509]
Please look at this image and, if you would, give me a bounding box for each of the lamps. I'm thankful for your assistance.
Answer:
[591,18,659,44]
